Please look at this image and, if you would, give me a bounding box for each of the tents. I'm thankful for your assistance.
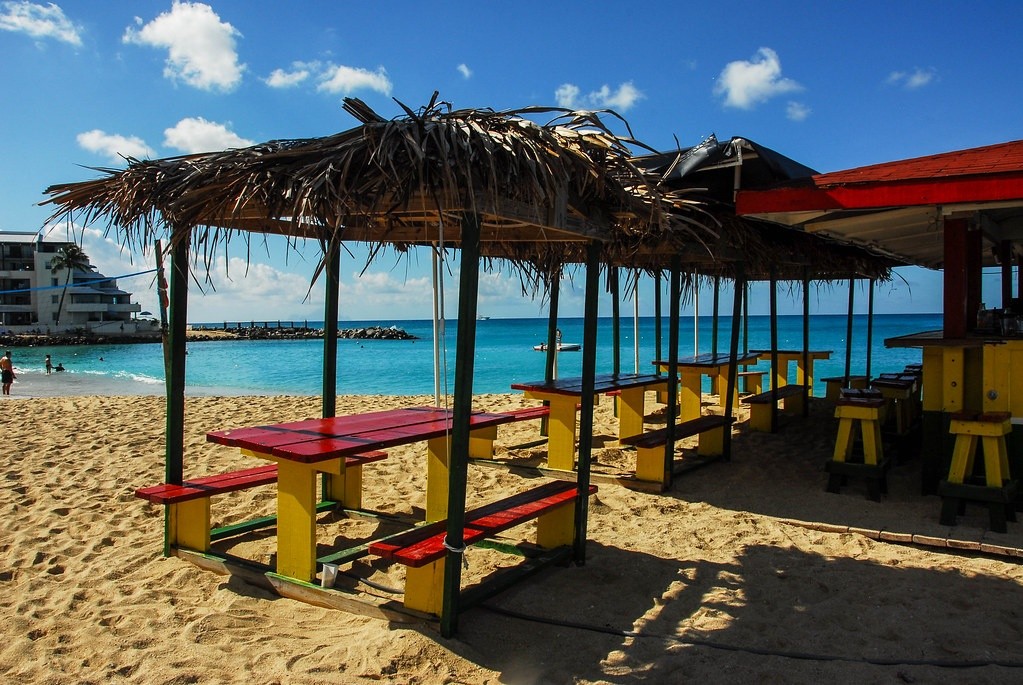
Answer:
[36,113,681,638]
[539,138,891,460]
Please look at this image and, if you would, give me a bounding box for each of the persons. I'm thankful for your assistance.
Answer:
[541,343,545,351]
[0,350,17,395]
[557,329,562,345]
[46,355,51,374]
[51,363,65,372]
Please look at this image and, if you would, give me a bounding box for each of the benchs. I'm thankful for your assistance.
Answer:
[136,363,1013,631]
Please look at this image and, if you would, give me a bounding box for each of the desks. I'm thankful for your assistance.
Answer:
[750,350,833,395]
[510,373,681,472]
[651,352,763,422]
[206,405,516,585]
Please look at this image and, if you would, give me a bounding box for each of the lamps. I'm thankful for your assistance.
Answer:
[991,241,1016,262]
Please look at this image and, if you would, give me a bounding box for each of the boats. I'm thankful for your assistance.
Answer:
[476,311,491,320]
[532,342,582,352]
